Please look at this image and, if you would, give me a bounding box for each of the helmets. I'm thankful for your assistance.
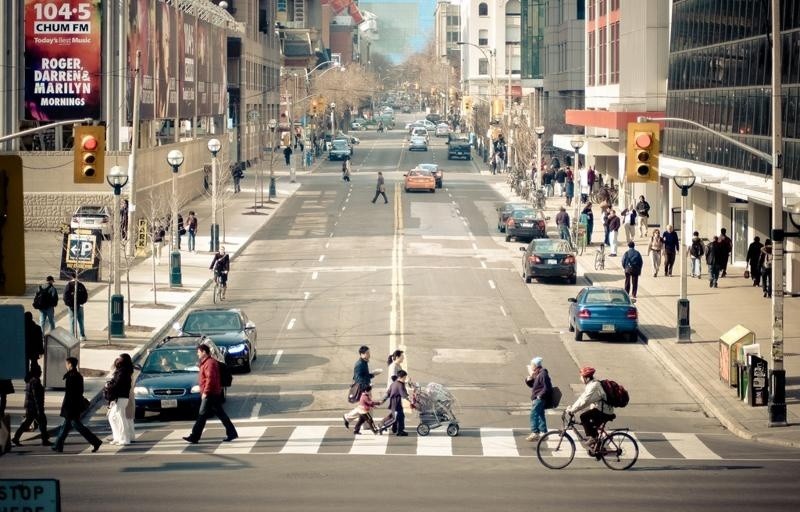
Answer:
[579,367,596,376]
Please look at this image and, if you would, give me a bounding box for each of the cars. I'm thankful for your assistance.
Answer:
[407,135,427,151]
[503,210,553,242]
[495,203,530,233]
[352,89,427,132]
[519,238,581,286]
[403,168,437,193]
[66,203,114,245]
[415,163,445,187]
[309,126,360,161]
[178,308,259,371]
[404,112,450,140]
[565,285,643,342]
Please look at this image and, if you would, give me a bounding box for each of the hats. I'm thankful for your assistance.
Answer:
[533,357,543,367]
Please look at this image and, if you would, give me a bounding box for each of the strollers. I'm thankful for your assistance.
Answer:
[406,377,466,439]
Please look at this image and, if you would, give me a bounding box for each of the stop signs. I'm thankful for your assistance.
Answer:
[0,477,61,512]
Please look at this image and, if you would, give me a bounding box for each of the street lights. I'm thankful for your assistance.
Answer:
[568,134,587,236]
[317,65,350,91]
[290,60,344,174]
[327,101,339,138]
[265,119,278,197]
[205,135,226,255]
[674,166,701,347]
[99,164,131,339]
[437,53,462,63]
[164,147,185,287]
[456,39,501,173]
[532,124,545,189]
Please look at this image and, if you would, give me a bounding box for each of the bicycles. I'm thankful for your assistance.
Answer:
[591,182,620,208]
[594,243,611,271]
[505,166,550,216]
[536,405,643,472]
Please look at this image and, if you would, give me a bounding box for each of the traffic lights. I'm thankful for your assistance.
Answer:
[627,126,663,182]
[70,125,109,185]
[430,88,437,97]
[462,99,473,112]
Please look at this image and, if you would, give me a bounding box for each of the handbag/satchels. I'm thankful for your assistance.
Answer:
[348,384,361,402]
[544,387,562,409]
[744,271,749,278]
[219,362,231,387]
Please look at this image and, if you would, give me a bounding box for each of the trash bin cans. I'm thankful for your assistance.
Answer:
[718,324,756,388]
[42,326,80,388]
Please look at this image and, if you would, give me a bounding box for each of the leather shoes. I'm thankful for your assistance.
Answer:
[51,447,63,452]
[92,439,102,452]
[223,437,236,441]
[183,437,198,443]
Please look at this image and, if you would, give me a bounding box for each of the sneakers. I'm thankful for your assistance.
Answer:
[526,431,548,441]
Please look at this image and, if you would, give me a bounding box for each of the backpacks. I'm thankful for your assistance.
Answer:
[33,284,53,309]
[690,242,704,258]
[599,380,629,407]
[763,254,773,269]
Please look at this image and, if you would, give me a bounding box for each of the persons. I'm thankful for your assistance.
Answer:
[343,345,409,435]
[283,133,305,165]
[371,171,389,204]
[342,155,351,181]
[488,132,507,175]
[0,270,136,453]
[564,367,615,446]
[120,198,129,239]
[209,246,230,300]
[182,345,238,444]
[232,163,243,194]
[149,210,198,264]
[524,356,550,441]
[528,153,773,300]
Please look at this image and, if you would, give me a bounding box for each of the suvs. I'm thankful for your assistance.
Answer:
[131,335,229,420]
[445,131,474,162]
